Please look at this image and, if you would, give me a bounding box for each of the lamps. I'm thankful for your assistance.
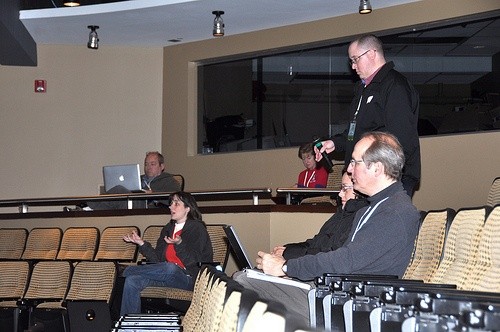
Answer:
[212,10,224,36]
[87,25,100,49]
[360,0,372,14]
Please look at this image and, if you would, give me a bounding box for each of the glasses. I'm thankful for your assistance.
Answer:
[351,160,371,167]
[340,186,354,192]
[350,50,376,64]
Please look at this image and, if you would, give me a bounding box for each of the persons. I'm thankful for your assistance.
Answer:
[118,191,214,316]
[139,151,181,192]
[314,34,421,201]
[290,142,329,188]
[231,131,421,329]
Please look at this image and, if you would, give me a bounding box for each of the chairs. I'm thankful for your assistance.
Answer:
[0,224,311,332]
[308,177,500,332]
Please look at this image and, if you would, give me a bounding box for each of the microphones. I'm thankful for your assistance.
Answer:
[311,135,334,168]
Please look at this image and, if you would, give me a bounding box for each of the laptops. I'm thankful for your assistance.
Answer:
[223,224,261,270]
[103,164,146,193]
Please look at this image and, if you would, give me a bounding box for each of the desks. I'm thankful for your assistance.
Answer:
[276,187,342,205]
[0,187,271,217]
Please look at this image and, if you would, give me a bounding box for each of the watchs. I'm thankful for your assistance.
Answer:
[281,259,288,276]
